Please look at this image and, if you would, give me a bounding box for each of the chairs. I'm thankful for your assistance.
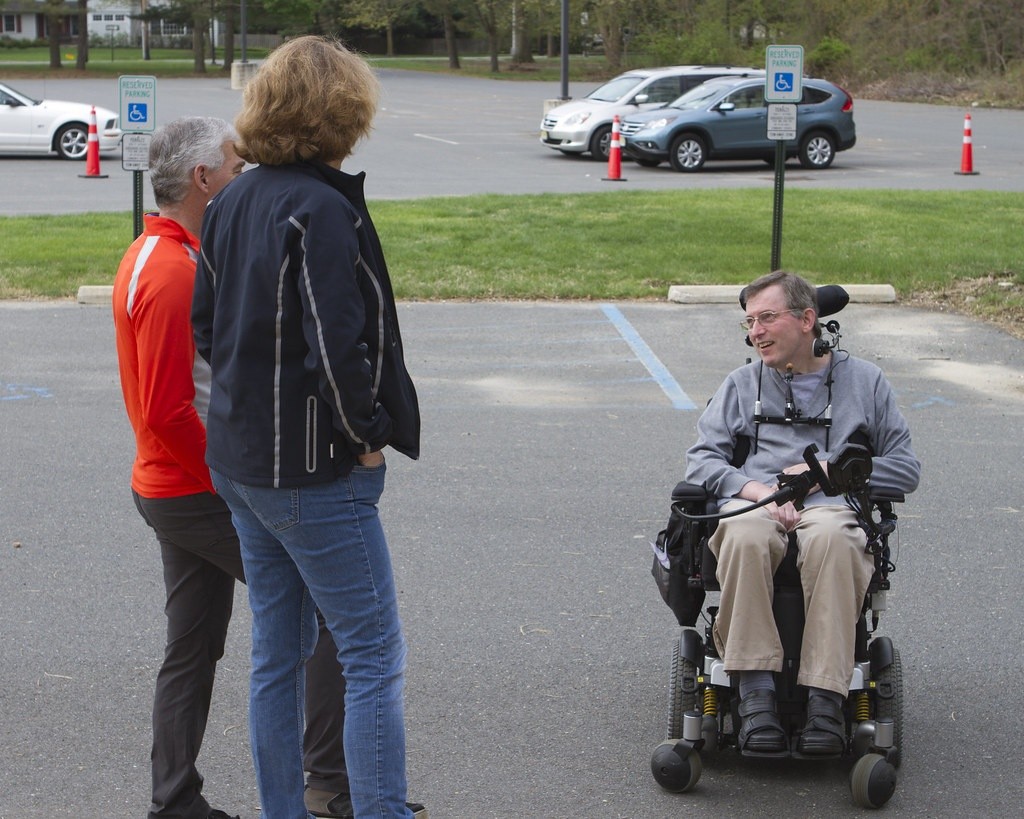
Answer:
[736,88,752,108]
[749,90,766,108]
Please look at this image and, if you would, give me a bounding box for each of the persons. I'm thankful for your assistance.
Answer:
[112,118,424,819]
[190,36,421,819]
[686,271,921,756]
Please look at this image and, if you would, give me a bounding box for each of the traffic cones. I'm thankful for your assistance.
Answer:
[602,114,629,183]
[78,105,110,178]
[953,112,982,175]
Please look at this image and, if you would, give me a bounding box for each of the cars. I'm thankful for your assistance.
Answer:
[0,82,122,163]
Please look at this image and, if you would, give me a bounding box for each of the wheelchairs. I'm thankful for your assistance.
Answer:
[653,356,907,810]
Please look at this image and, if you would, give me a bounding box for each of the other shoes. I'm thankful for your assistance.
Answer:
[800,694,846,753]
[303,784,428,819]
[204,809,240,819]
[738,688,786,752]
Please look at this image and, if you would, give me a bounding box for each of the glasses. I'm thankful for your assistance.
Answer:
[740,308,804,330]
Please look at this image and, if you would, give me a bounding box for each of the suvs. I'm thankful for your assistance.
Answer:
[619,72,857,173]
[541,66,755,167]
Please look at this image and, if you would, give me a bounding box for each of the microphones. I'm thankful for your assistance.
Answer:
[812,320,840,360]
